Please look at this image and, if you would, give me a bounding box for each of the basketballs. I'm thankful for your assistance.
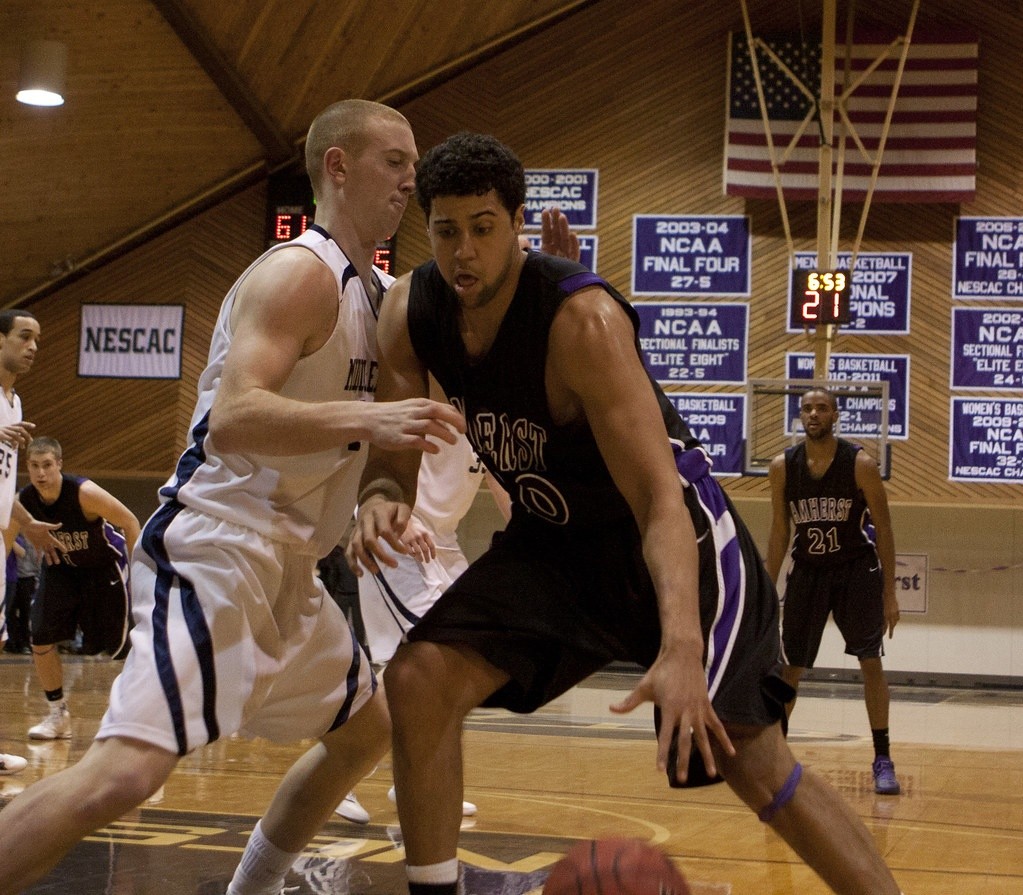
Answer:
[539,837,691,895]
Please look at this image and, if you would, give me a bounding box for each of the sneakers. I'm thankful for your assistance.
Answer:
[27,709,73,739]
[293,848,351,895]
[335,789,370,823]
[387,784,477,816]
[0,752,28,774]
[871,755,900,794]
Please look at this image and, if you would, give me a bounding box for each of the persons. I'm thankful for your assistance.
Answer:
[335,420,512,825]
[0,99,580,895]
[344,131,898,895]
[766,387,901,795]
[0,309,65,775]
[13,435,140,741]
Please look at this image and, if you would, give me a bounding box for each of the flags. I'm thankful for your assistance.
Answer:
[722,31,978,203]
